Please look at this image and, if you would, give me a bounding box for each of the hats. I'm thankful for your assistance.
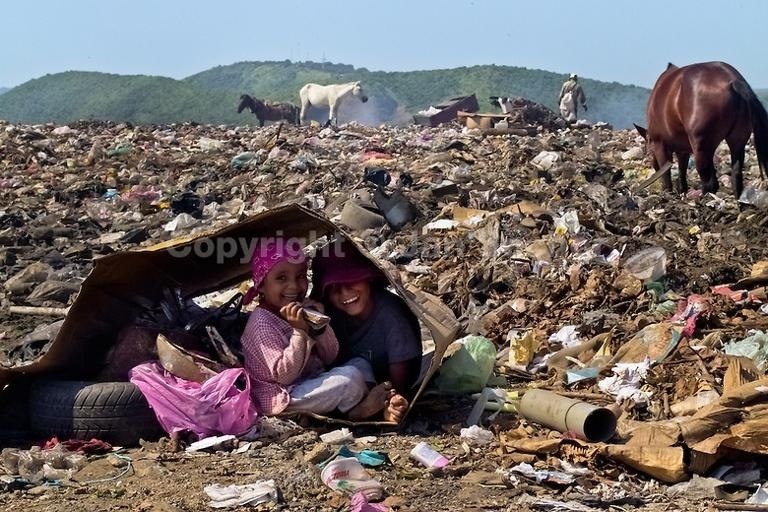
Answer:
[308,243,385,301]
[241,233,305,305]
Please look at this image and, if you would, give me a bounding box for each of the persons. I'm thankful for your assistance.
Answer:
[239,235,396,420]
[307,240,421,421]
[558,72,587,123]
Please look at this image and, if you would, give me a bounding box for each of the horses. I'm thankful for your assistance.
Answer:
[237,94,301,128]
[299,80,369,128]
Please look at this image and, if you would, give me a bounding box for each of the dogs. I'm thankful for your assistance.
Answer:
[632,60,768,200]
